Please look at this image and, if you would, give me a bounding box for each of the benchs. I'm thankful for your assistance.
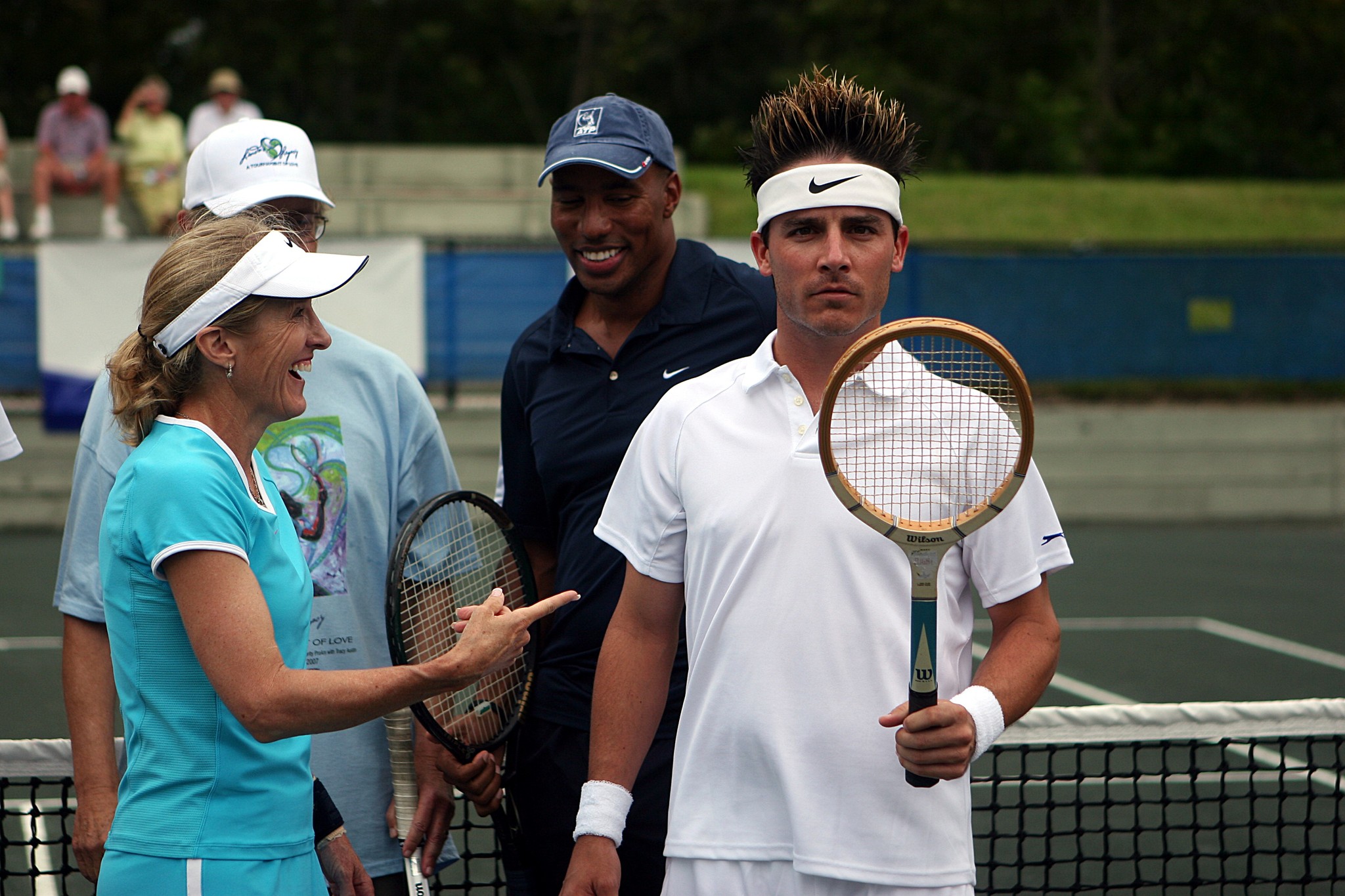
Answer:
[0,145,706,251]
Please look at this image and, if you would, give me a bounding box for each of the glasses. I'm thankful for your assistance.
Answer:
[264,210,329,243]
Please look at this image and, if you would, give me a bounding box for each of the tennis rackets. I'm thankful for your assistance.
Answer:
[815,314,1037,792]
[380,707,432,896]
[382,490,544,896]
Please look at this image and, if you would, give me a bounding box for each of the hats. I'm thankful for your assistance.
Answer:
[207,68,242,97]
[56,66,90,96]
[182,117,334,218]
[151,229,369,361]
[537,93,678,188]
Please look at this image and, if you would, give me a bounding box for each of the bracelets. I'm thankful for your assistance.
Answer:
[949,685,1006,763]
[465,698,509,722]
[573,779,635,849]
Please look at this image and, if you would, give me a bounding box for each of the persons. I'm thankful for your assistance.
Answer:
[559,64,1072,896]
[53,119,481,896]
[0,68,261,245]
[423,93,779,896]
[96,209,583,896]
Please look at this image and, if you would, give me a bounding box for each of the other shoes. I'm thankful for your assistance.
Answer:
[28,204,53,239]
[0,217,27,247]
[99,205,129,240]
